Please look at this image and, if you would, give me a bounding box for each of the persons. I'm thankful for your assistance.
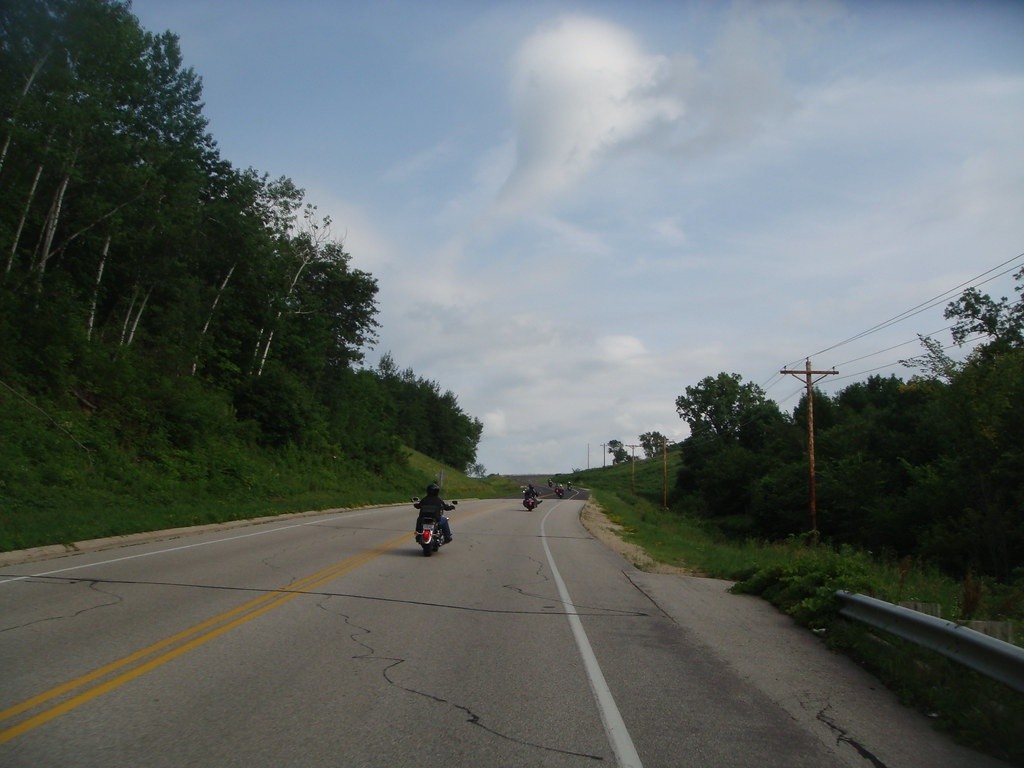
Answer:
[414,482,455,543]
[547,477,572,495]
[524,483,543,504]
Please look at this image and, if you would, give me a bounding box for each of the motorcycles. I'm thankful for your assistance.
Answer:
[411,496,458,557]
[522,489,543,511]
[548,478,572,498]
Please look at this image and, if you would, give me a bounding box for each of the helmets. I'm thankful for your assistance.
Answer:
[427,483,440,494]
[529,482,535,488]
[560,484,563,487]
[568,481,570,483]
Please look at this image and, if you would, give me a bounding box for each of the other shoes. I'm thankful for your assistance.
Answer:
[444,536,452,543]
